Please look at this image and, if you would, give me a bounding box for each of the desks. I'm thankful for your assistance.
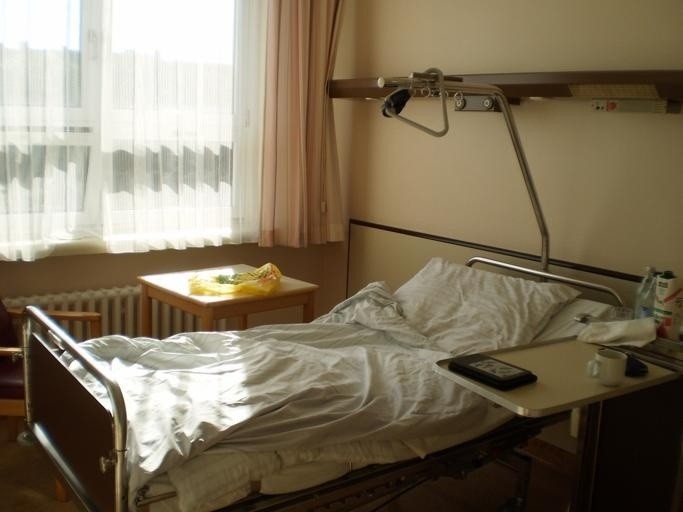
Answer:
[431,333,678,512]
[136,263,320,338]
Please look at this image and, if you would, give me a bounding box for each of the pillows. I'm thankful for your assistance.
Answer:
[388,256,581,358]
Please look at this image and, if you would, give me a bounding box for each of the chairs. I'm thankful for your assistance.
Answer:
[1,298,102,418]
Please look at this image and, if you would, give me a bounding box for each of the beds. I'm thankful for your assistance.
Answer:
[18,255,630,512]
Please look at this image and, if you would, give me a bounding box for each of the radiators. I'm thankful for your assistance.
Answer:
[2,285,228,343]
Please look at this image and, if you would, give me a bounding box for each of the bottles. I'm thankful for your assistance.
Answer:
[637,264,680,337]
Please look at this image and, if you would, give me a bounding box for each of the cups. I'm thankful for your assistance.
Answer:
[586,349,627,387]
